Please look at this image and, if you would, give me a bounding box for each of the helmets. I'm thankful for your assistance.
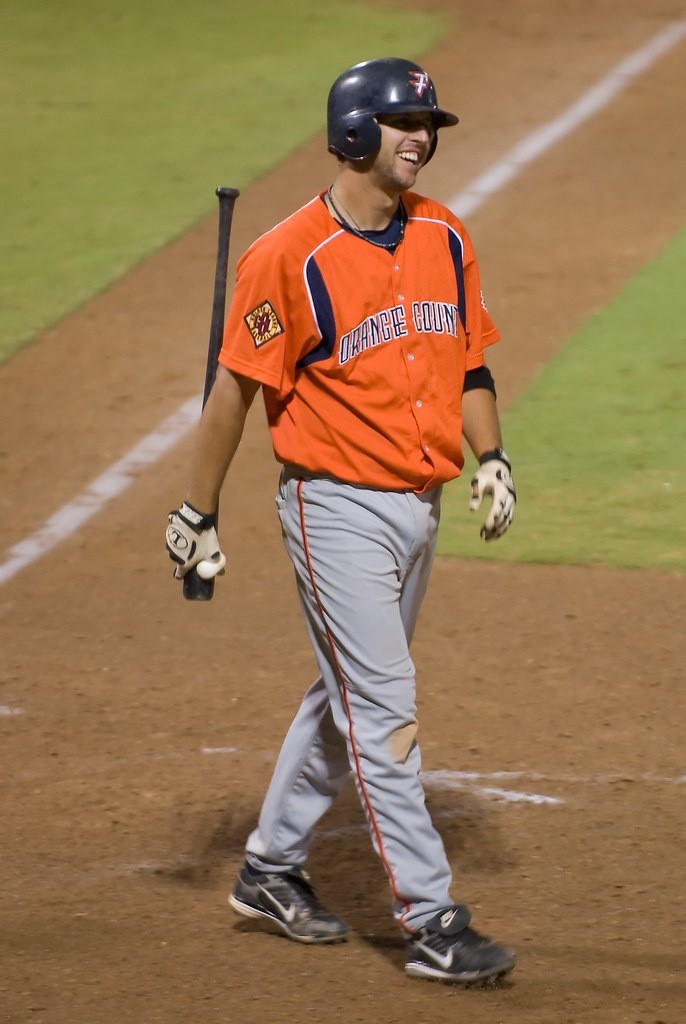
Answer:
[327,56,459,165]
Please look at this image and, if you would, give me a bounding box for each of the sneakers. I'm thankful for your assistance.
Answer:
[227,862,348,944]
[405,904,515,983]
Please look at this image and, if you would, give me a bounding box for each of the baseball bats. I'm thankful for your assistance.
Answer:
[182,186,240,601]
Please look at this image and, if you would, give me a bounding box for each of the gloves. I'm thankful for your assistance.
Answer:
[469,458,516,539]
[165,501,227,579]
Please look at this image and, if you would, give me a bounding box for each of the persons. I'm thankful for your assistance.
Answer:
[164,57,518,980]
